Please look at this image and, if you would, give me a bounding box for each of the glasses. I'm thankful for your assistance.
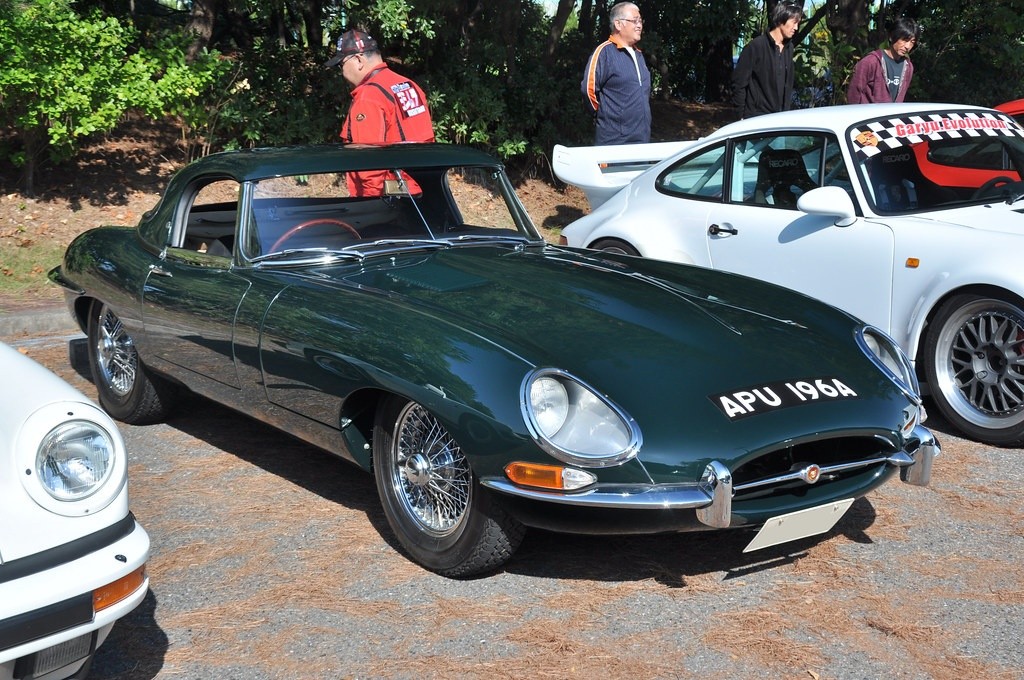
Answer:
[618,18,642,25]
[338,53,363,73]
[787,3,805,18]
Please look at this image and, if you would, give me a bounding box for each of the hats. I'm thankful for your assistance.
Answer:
[323,30,377,67]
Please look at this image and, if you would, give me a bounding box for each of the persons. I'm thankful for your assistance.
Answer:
[848,18,918,104]
[581,1,651,145]
[324,29,435,197]
[730,0,805,122]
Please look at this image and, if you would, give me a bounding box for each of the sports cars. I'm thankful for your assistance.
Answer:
[49,142,941,583]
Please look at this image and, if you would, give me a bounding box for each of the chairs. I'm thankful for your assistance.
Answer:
[886,146,972,207]
[755,149,818,211]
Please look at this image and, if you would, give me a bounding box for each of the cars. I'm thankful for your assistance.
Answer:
[552,103,1024,449]
[0,345,152,680]
